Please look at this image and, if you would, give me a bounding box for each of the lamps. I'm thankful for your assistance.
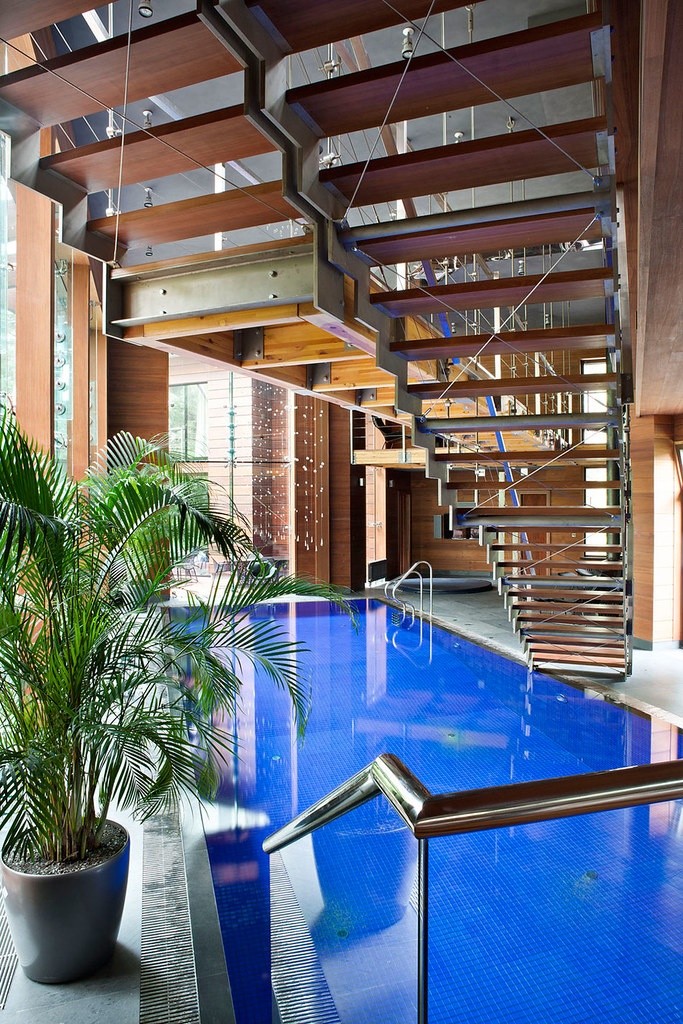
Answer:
[145,245,153,257]
[142,110,153,128]
[454,131,465,144]
[401,27,415,58]
[138,0,154,19]
[545,313,551,325]
[451,322,457,333]
[143,187,154,208]
[517,259,524,275]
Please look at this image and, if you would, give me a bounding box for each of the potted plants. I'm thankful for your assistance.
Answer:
[0,390,360,984]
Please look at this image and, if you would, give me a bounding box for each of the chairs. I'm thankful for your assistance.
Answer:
[517,557,614,616]
[176,551,277,591]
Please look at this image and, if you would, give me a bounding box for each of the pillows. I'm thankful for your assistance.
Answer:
[251,563,270,576]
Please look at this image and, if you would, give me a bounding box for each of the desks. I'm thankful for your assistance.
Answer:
[224,556,289,586]
